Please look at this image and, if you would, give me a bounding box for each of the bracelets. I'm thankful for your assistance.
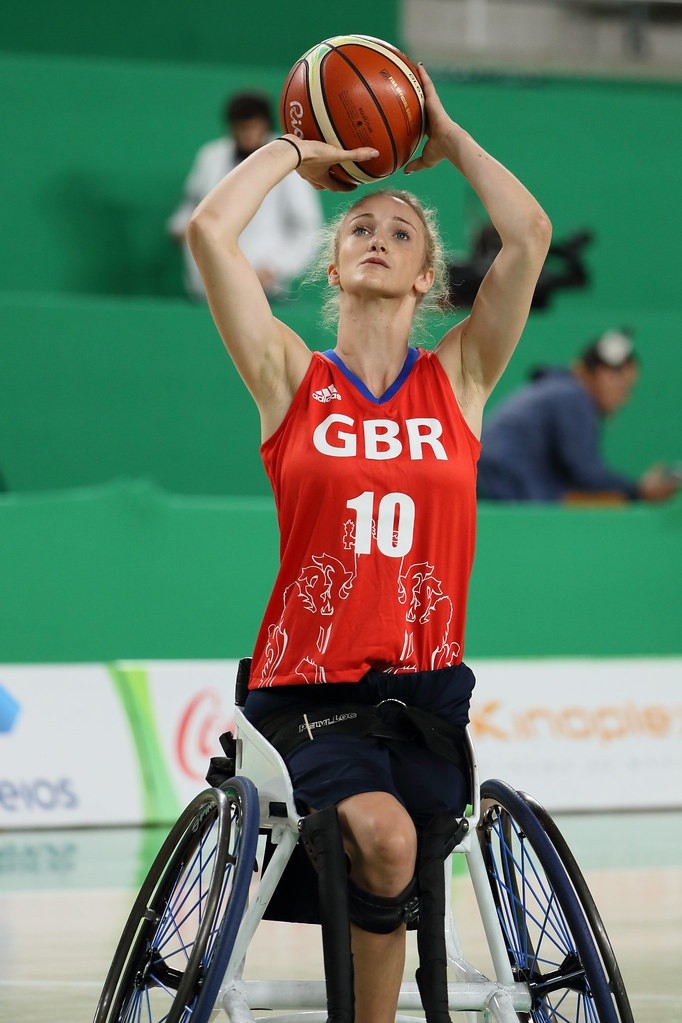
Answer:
[275,138,302,170]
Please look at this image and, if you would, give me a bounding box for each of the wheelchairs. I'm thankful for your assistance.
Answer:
[80,654,637,1023]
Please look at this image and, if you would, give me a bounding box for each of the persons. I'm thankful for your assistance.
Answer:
[476,323,682,504]
[190,60,551,1023]
[166,88,324,303]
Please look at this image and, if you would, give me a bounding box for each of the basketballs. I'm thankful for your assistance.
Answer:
[281,34,428,186]
